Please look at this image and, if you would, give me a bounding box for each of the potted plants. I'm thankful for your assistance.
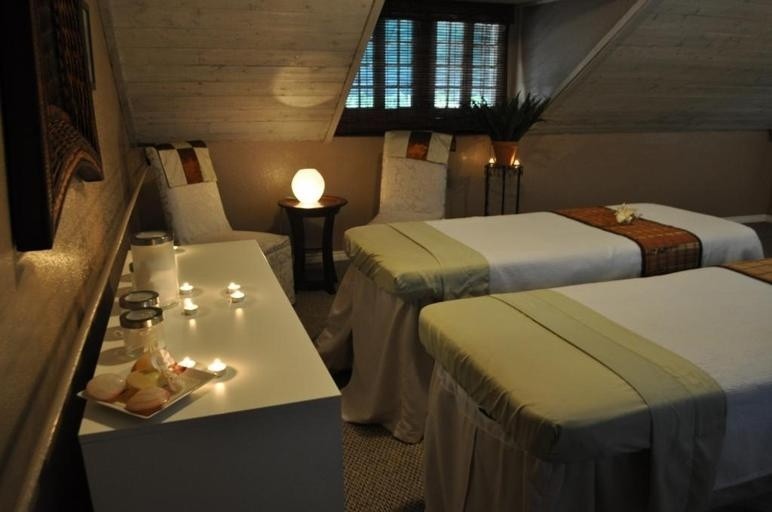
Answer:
[459,90,550,169]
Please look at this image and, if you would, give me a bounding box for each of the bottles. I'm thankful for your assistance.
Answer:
[119,289,160,308]
[119,308,166,358]
[130,231,180,307]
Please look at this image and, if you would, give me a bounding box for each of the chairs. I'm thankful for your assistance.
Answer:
[147,140,298,308]
[369,132,452,226]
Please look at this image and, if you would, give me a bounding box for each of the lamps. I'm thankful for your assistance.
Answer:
[292,168,324,208]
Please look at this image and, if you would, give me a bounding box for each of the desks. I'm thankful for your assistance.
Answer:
[278,194,349,294]
[78,236,344,512]
[482,161,521,215]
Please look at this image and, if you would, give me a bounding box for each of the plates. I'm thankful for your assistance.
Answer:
[76,358,217,420]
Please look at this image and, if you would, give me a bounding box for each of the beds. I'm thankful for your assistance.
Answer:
[315,201,761,445]
[415,257,772,512]
